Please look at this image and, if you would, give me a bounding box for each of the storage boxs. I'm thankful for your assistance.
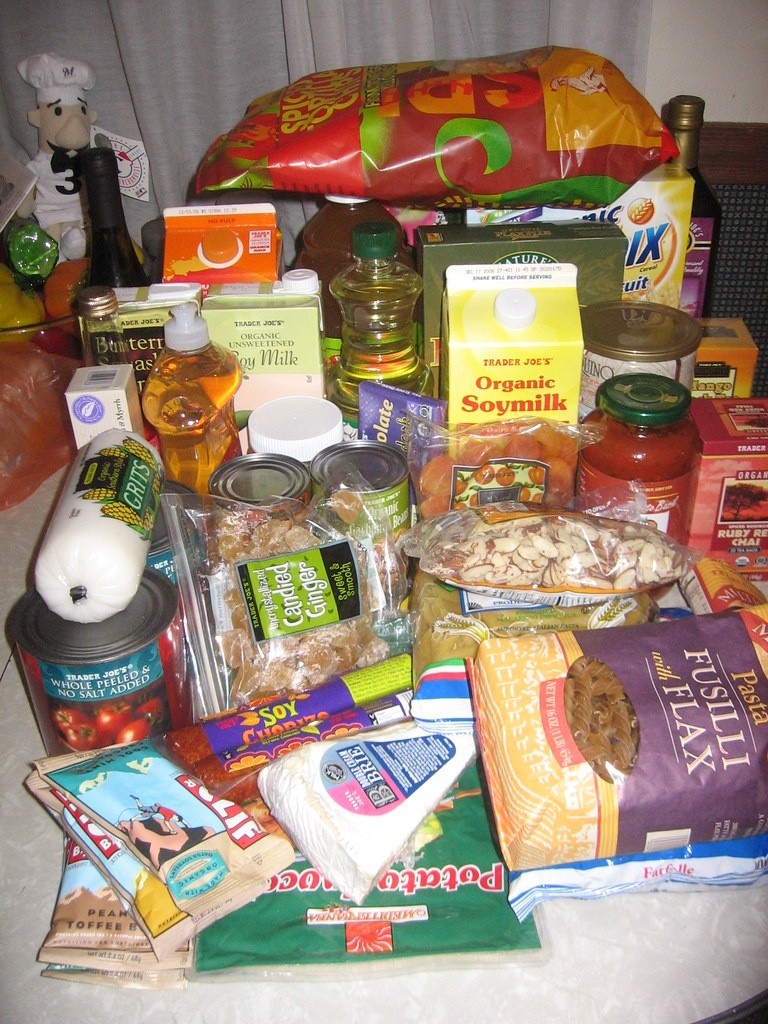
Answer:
[414,162,696,424]
[692,316,758,397]
[686,398,768,582]
[65,365,144,445]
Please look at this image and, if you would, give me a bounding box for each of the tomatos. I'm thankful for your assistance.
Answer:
[50,698,163,750]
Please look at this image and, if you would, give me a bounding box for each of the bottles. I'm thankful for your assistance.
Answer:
[283,193,435,444]
[78,146,151,286]
[666,95,723,318]
[141,302,243,494]
[76,286,131,364]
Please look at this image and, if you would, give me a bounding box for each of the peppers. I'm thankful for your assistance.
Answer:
[0,263,46,341]
[46,259,92,335]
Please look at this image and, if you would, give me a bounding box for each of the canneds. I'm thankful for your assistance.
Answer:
[309,441,410,540]
[579,300,700,422]
[146,479,209,619]
[577,375,698,602]
[8,567,191,757]
[209,453,312,541]
